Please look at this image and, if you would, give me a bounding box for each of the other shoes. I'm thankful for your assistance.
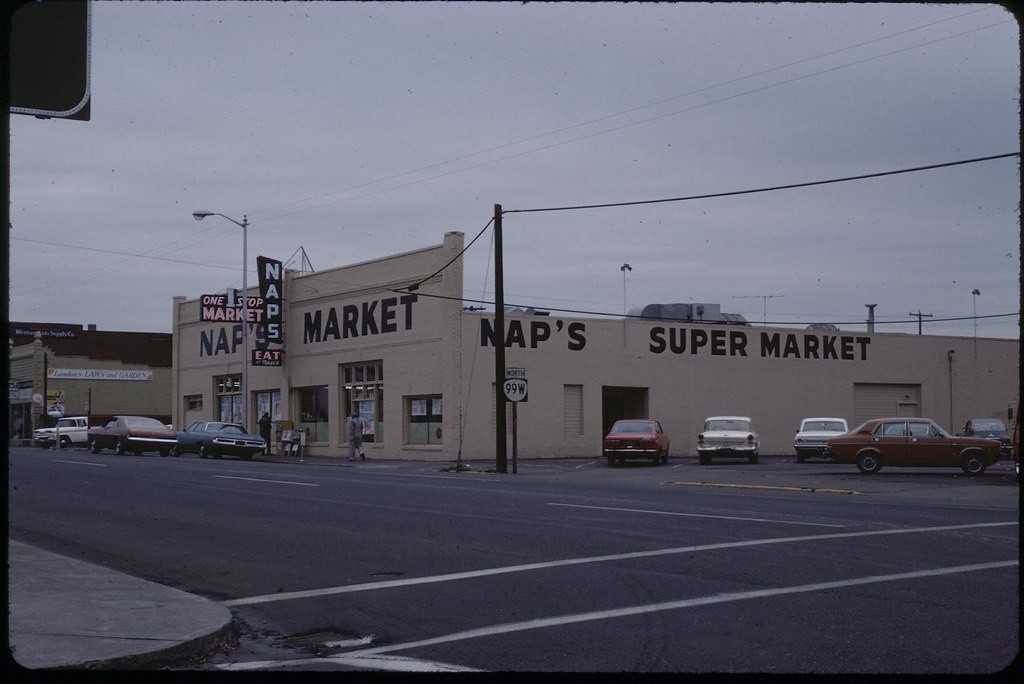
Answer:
[361,453,365,461]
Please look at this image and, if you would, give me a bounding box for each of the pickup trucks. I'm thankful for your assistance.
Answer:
[31,416,104,449]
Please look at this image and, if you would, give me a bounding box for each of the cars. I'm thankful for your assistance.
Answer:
[827,417,1003,475]
[602,420,670,466]
[698,415,760,466]
[176,420,268,461]
[963,418,1014,458]
[795,417,850,466]
[86,415,179,458]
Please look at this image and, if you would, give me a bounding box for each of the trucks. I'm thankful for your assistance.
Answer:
[1007,399,1021,475]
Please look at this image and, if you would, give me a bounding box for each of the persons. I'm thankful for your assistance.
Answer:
[347,412,366,461]
[260,412,272,455]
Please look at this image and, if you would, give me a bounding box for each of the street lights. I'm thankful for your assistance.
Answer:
[193,207,250,433]
